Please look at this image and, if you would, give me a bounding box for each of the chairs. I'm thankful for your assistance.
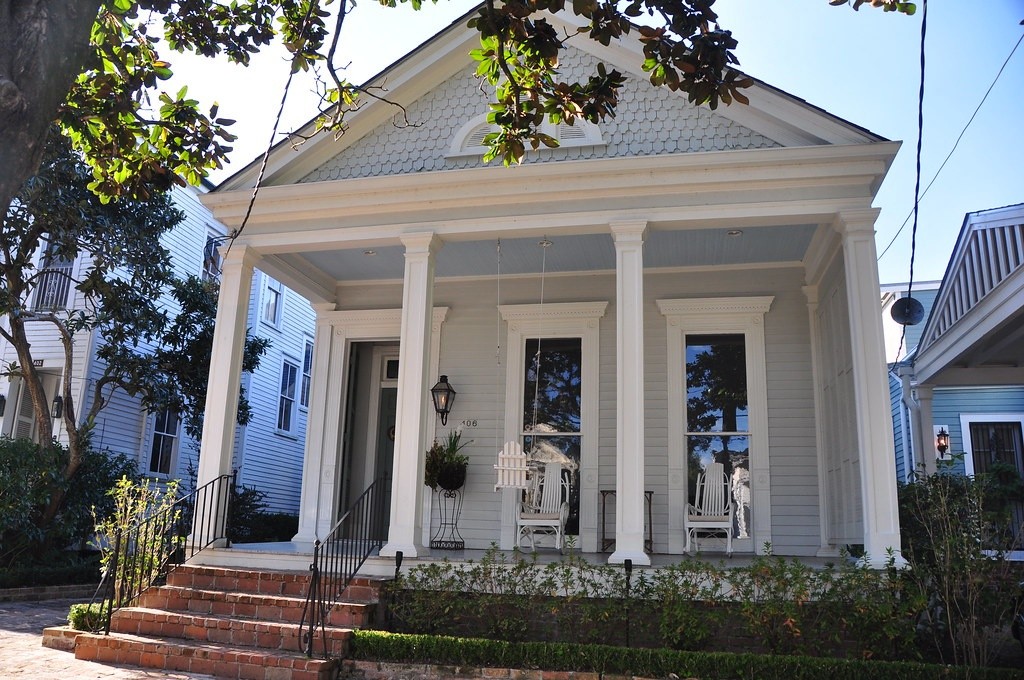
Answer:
[514,461,570,556]
[682,462,736,559]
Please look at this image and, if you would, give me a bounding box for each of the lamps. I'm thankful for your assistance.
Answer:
[50,395,63,419]
[937,427,949,459]
[430,374,457,426]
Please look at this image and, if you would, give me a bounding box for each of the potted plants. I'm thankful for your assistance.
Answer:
[425,429,476,491]
